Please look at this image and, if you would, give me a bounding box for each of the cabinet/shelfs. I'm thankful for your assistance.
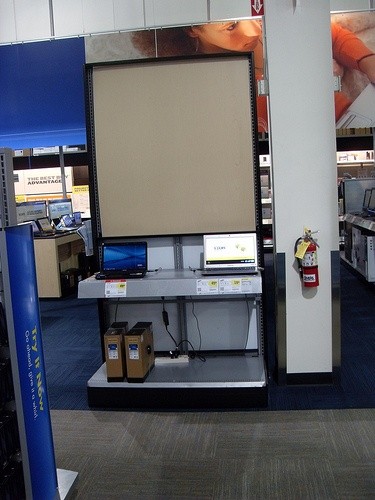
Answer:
[339,178,375,294]
[261,161,375,252]
[77,233,271,412]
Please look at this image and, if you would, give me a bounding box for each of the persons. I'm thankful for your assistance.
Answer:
[130,15,375,133]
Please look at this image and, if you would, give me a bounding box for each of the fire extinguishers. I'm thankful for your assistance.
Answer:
[300,230,320,288]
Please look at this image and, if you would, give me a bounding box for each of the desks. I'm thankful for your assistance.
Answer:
[34,227,84,300]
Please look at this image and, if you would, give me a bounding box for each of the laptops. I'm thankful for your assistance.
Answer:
[200,233,257,275]
[95,241,148,281]
[24,212,84,236]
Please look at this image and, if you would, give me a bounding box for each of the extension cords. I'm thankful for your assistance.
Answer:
[155,354,189,363]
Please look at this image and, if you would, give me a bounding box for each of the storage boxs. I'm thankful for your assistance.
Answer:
[352,227,375,282]
